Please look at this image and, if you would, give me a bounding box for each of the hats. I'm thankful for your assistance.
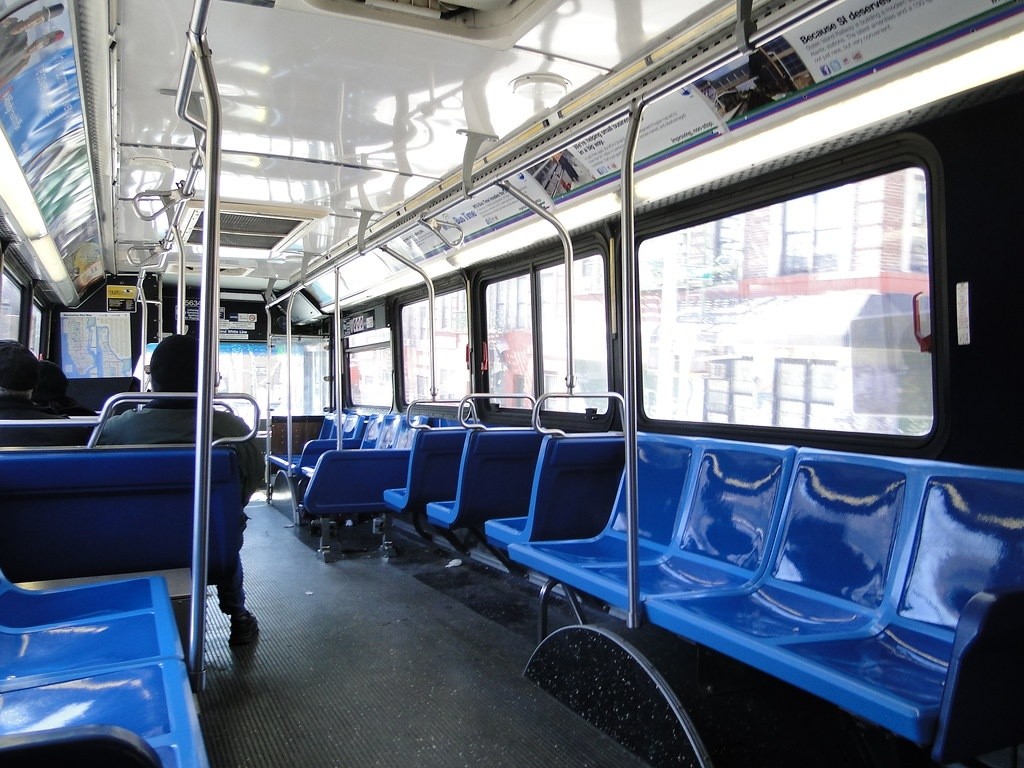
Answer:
[0,340,41,392]
[150,334,197,392]
[39,359,69,392]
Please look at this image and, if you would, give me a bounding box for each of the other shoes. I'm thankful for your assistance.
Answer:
[228,610,260,647]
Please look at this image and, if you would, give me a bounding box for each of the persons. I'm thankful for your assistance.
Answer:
[0,340,100,448]
[96,333,265,645]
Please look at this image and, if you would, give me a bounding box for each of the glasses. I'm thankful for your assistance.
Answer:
[144,365,151,373]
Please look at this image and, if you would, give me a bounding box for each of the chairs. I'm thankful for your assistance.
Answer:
[269,409,627,579]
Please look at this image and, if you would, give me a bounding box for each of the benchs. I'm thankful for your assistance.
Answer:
[0,376,261,768]
[507,434,1024,768]
